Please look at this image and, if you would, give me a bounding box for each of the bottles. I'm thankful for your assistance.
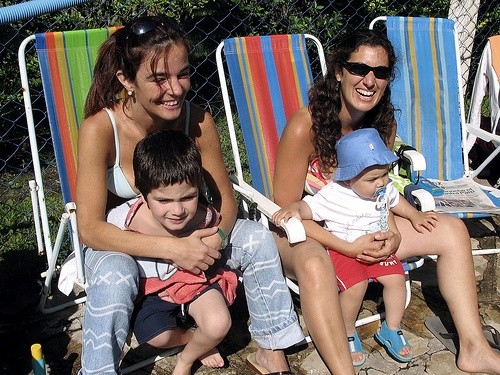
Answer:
[31,343,46,375]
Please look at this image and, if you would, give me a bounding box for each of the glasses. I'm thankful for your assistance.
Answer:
[341,61,393,80]
[127,15,180,37]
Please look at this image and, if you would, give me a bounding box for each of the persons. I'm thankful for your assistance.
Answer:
[75,16,304,375]
[106,130,238,375]
[273,28,500,375]
[272,127,440,366]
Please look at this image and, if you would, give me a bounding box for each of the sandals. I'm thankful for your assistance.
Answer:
[346,329,365,366]
[374,320,413,363]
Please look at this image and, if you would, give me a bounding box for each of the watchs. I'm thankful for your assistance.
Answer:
[216,228,228,250]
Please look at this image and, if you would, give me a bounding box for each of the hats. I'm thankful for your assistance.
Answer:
[331,128,400,181]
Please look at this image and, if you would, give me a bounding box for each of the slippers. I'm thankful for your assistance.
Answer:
[245,352,296,375]
[424,314,461,354]
[480,324,500,349]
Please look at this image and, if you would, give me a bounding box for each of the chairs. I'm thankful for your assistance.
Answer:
[17,16,500,375]
